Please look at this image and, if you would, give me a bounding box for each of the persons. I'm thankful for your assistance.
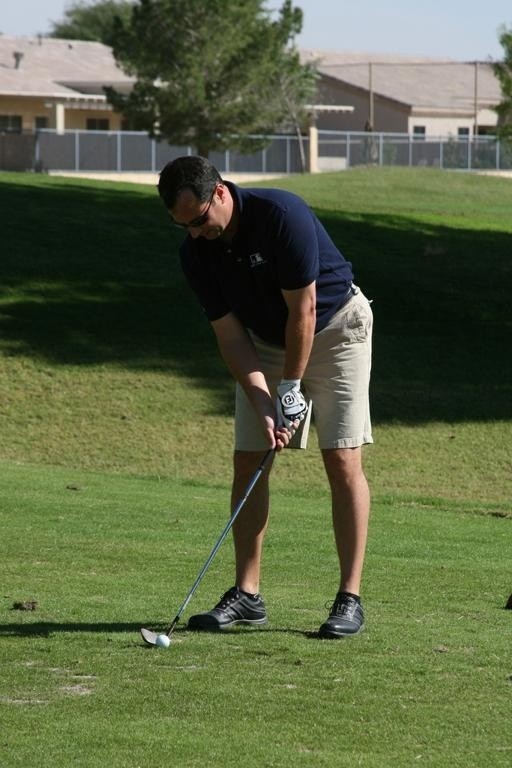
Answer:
[156,156,373,640]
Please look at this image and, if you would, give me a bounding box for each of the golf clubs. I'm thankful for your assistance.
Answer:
[140,428,293,644]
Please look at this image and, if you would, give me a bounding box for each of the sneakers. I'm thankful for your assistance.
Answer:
[190,585,267,628]
[319,592,366,638]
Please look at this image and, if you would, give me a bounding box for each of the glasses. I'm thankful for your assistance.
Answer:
[169,187,217,231]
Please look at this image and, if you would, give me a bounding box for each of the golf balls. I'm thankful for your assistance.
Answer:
[156,634,170,648]
